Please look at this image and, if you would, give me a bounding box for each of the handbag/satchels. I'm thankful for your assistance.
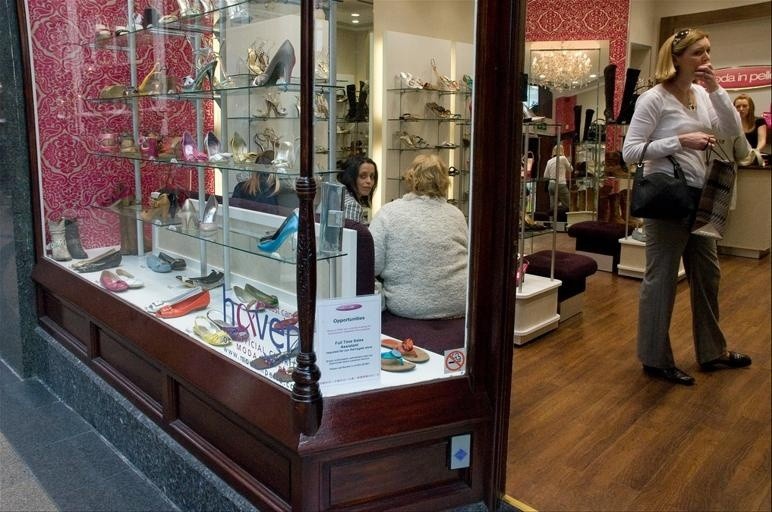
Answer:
[630,164,689,218]
[691,159,735,240]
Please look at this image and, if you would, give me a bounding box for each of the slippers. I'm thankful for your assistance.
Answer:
[382,338,429,363]
[382,350,416,372]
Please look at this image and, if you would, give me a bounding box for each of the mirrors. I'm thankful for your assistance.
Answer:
[334,1,375,169]
[518,39,611,258]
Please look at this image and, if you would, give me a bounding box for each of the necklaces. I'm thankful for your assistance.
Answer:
[674,89,696,112]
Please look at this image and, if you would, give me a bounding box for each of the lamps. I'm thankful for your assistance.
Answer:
[530,41,592,92]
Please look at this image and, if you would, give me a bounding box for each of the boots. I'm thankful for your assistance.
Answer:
[65,216,88,259]
[356,80,369,122]
[344,84,357,122]
[48,218,71,261]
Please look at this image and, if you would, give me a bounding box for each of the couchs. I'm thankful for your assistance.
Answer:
[521,249,598,323]
[567,221,638,275]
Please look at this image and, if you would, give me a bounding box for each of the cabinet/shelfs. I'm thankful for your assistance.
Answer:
[520,121,565,241]
[388,88,473,182]
[81,0,348,265]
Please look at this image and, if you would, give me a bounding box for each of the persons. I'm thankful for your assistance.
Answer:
[543,143,574,213]
[733,94,766,151]
[367,152,469,320]
[313,154,378,224]
[620,28,753,385]
[230,149,299,208]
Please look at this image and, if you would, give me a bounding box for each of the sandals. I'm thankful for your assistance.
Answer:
[399,73,460,176]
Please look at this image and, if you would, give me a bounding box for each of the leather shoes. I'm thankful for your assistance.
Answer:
[71,248,299,382]
[642,364,695,386]
[698,351,752,371]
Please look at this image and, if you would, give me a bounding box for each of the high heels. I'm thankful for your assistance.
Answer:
[251,40,296,86]
[141,193,171,223]
[516,258,529,286]
[200,196,218,232]
[257,213,298,251]
[167,192,176,219]
[175,60,218,92]
[182,198,200,229]
[98,130,296,165]
[252,94,287,118]
[92,0,222,41]
[246,46,270,74]
